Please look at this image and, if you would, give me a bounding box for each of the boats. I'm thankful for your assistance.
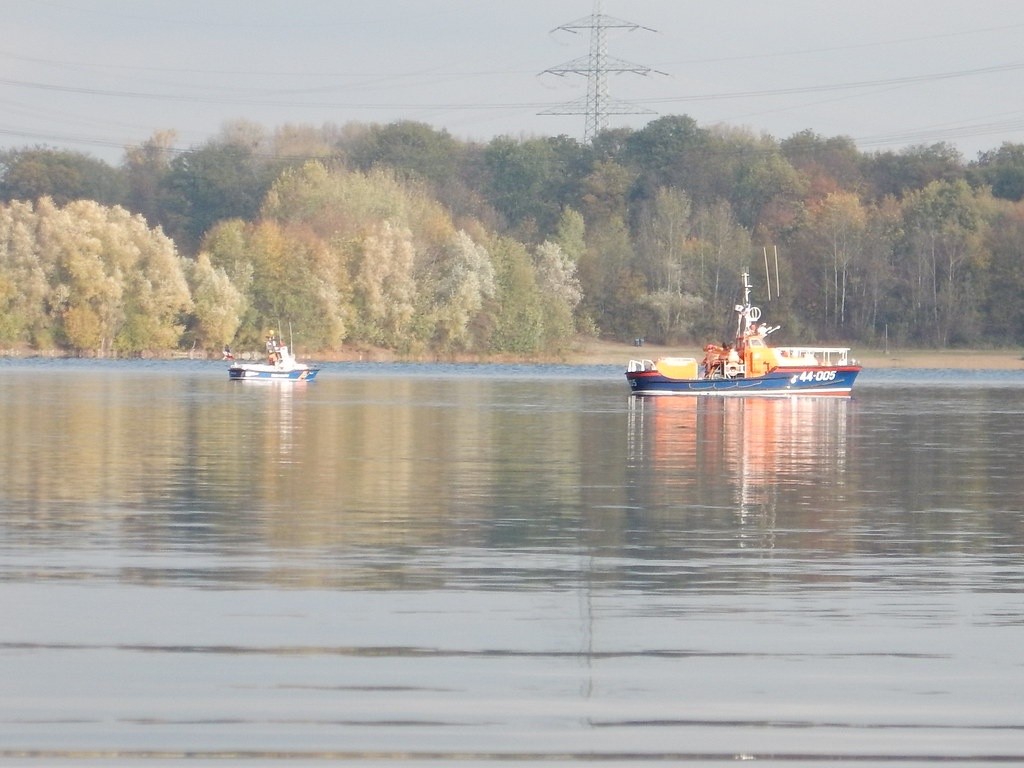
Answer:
[624,273,863,398]
[228,318,321,381]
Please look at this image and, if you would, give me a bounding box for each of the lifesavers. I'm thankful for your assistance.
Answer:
[727,362,739,376]
